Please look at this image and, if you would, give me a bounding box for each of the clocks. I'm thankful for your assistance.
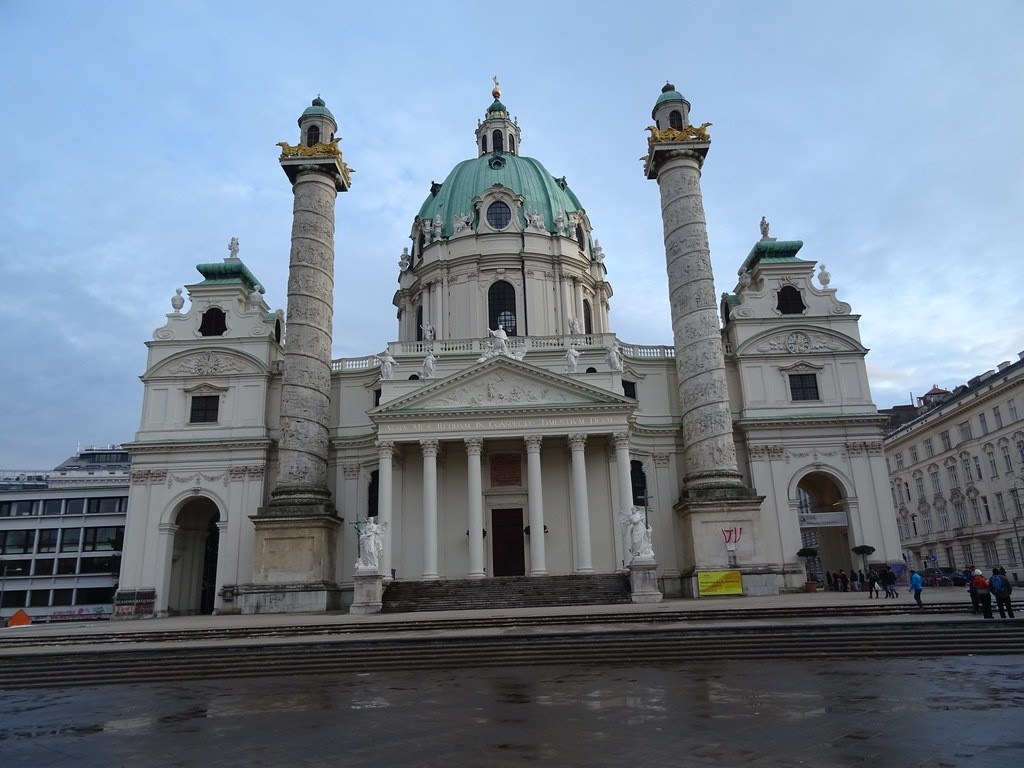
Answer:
[786,330,811,355]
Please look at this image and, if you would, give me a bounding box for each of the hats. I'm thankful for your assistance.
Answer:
[992,568,1002,575]
[1000,566,1006,575]
[974,569,982,576]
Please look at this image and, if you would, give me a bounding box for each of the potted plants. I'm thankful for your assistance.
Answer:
[797,548,819,592]
[851,545,875,591]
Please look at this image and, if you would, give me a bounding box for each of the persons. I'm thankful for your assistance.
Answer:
[398,210,472,266]
[527,204,605,262]
[483,325,527,361]
[423,351,440,378]
[618,507,646,556]
[376,352,399,380]
[562,345,585,372]
[420,322,434,340]
[228,237,240,258]
[569,317,582,345]
[606,342,621,370]
[360,517,389,567]
[760,216,770,235]
[827,565,1014,619]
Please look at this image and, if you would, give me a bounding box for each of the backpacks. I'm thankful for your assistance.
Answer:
[990,574,1005,595]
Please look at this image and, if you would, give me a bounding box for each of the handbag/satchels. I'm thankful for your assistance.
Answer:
[976,589,989,601]
[874,582,882,591]
[965,582,976,594]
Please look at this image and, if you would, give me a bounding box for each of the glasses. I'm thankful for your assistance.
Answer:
[966,567,970,568]
